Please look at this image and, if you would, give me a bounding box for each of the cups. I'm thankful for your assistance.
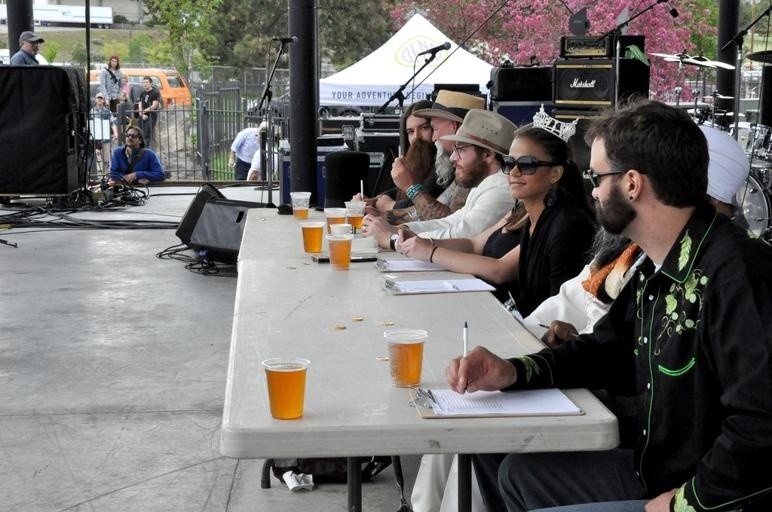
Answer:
[290,191,312,219]
[300,221,325,253]
[344,201,367,230]
[383,327,429,387]
[323,207,348,237]
[261,356,311,422]
[329,223,353,238]
[325,234,354,271]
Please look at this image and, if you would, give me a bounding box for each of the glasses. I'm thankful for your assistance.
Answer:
[501,155,559,175]
[125,134,140,137]
[451,144,473,155]
[583,170,647,186]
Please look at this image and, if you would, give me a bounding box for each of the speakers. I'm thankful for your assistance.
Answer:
[189,200,277,266]
[550,108,608,123]
[175,183,226,247]
[551,56,648,111]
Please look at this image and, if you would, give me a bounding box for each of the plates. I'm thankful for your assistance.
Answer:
[326,234,354,241]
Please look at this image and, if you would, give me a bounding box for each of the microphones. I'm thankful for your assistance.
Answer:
[662,2,682,17]
[272,34,302,45]
[414,41,453,56]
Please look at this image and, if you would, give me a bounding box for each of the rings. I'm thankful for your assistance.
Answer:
[363,226,367,232]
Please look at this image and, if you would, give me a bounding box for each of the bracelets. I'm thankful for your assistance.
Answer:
[429,246,439,264]
[405,183,423,198]
[428,237,435,246]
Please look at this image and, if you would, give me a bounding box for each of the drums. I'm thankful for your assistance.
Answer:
[744,157,770,183]
[729,123,772,153]
[737,170,771,240]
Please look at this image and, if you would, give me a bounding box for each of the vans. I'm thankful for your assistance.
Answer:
[87,67,192,111]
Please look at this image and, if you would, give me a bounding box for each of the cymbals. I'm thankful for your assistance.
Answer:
[711,61,735,70]
[704,95,734,99]
[746,50,771,64]
[663,58,717,69]
[648,53,680,58]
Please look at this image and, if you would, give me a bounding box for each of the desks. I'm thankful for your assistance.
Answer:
[237,208,446,270]
[218,260,621,512]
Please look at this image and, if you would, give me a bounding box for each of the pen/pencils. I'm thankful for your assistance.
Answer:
[360,180,364,200]
[464,322,468,357]
[539,324,580,338]
[398,144,401,158]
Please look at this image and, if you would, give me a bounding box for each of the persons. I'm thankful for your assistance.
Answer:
[410,125,750,512]
[118,75,134,141]
[396,104,596,319]
[247,128,278,181]
[366,90,486,226]
[446,98,772,512]
[351,99,455,212]
[10,30,44,65]
[360,106,521,253]
[228,122,269,180]
[97,55,123,116]
[89,91,118,180]
[110,125,165,186]
[138,75,161,146]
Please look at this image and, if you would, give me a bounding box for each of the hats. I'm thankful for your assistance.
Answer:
[20,30,44,43]
[96,93,104,98]
[440,108,519,155]
[413,89,486,123]
[699,125,750,204]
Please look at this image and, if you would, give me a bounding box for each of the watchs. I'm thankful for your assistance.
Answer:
[390,233,399,251]
